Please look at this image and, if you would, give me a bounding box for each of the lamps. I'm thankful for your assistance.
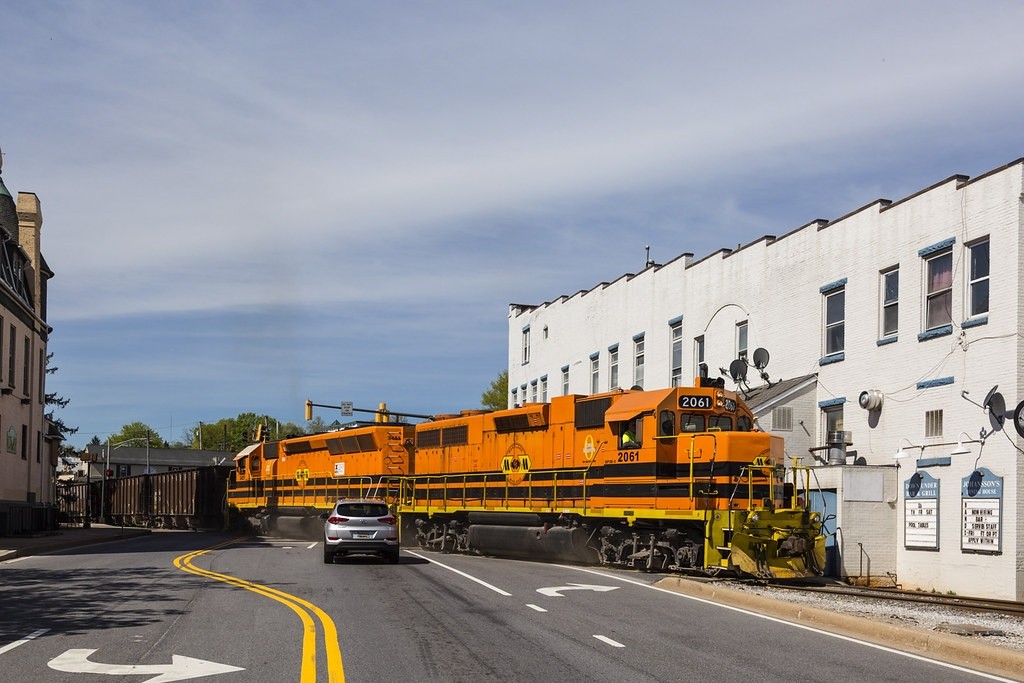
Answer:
[891,437,914,459]
[950,432,973,455]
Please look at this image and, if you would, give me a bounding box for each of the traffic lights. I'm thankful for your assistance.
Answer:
[106,470,114,477]
[256,424,270,442]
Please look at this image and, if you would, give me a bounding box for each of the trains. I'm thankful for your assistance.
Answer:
[225,362,828,587]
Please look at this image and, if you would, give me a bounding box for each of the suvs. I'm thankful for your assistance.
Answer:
[320,497,400,564]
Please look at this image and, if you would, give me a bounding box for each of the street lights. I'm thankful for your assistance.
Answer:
[107,438,147,480]
